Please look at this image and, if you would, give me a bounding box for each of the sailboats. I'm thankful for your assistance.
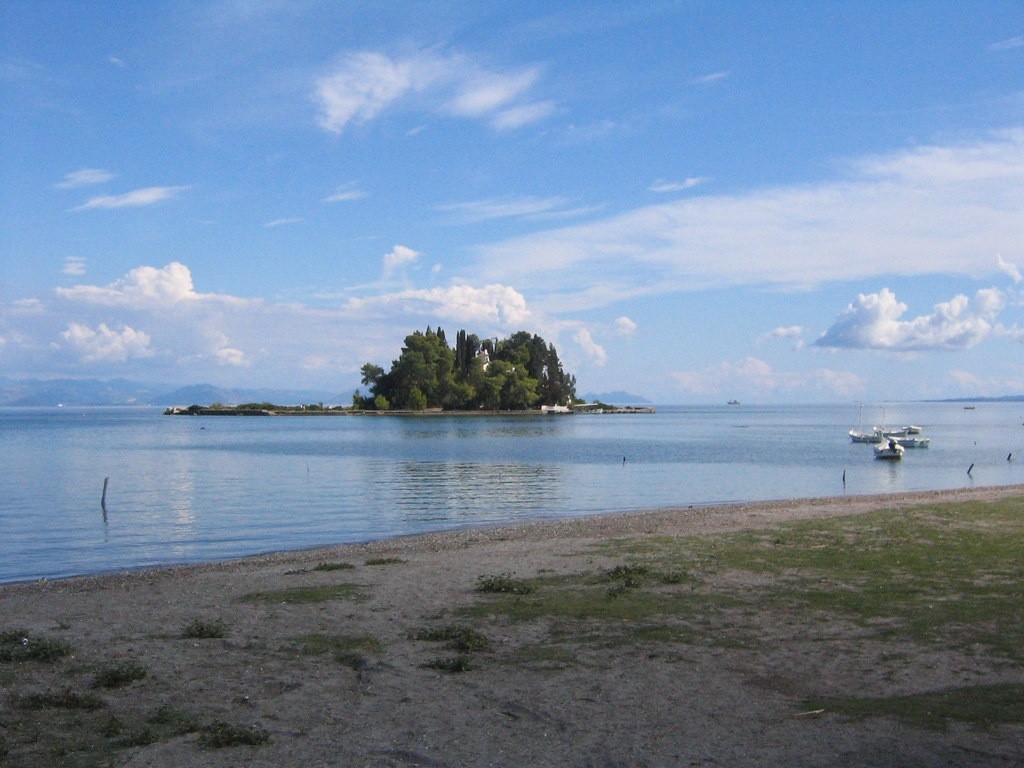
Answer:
[848,405,884,445]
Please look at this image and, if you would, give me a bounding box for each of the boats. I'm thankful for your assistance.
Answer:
[872,424,911,437]
[894,435,931,449]
[873,436,906,459]
[728,399,739,405]
[901,424,922,435]
